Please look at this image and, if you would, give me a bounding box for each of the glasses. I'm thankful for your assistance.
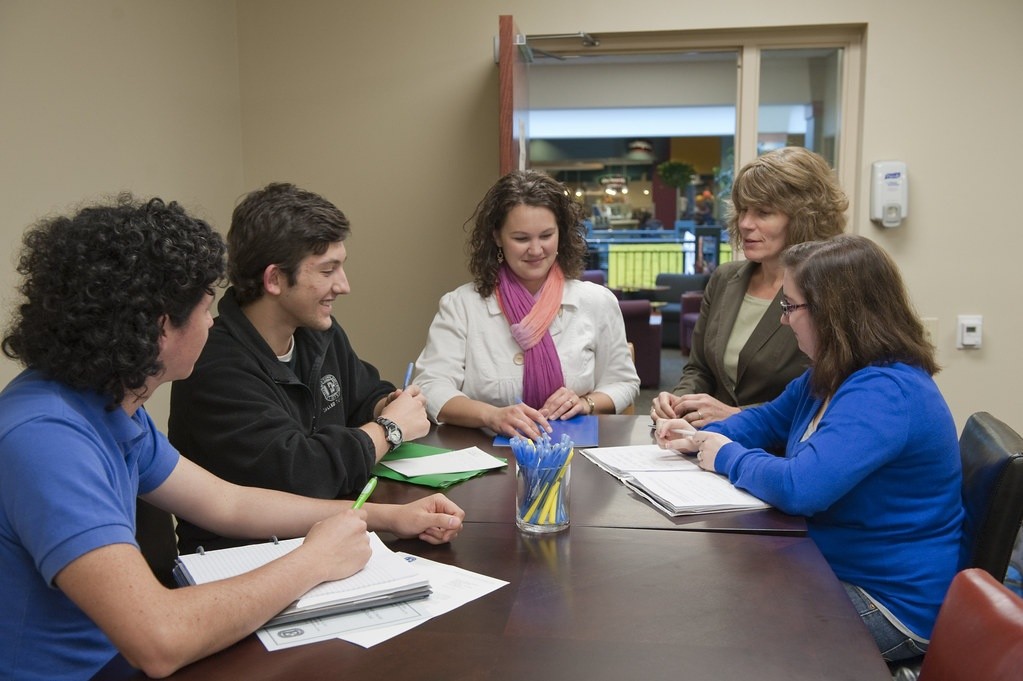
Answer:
[779,299,812,317]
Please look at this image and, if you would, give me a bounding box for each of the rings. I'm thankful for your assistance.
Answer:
[650,409,655,413]
[699,451,703,459]
[697,411,703,419]
[569,400,573,404]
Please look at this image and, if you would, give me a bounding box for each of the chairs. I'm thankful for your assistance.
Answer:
[887,410,1023,681]
[898,567,1023,681]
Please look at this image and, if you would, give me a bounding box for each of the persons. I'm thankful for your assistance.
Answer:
[654,234,966,661]
[168,182,430,553]
[0,195,466,681]
[650,146,848,433]
[411,169,643,445]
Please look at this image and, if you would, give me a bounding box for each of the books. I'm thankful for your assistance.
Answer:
[174,528,432,627]
[492,415,599,448]
[580,443,770,517]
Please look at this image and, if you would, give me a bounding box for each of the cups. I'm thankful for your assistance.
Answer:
[515,462,571,535]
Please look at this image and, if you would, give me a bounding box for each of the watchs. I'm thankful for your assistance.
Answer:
[583,395,594,414]
[374,417,403,452]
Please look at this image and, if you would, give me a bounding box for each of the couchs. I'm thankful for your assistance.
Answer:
[575,271,710,388]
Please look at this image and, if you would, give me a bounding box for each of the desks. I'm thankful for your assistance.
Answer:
[76,415,895,681]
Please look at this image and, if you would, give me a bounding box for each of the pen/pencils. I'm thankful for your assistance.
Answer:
[352,476,378,509]
[648,424,694,435]
[514,396,552,442]
[403,362,414,391]
[509,432,574,525]
